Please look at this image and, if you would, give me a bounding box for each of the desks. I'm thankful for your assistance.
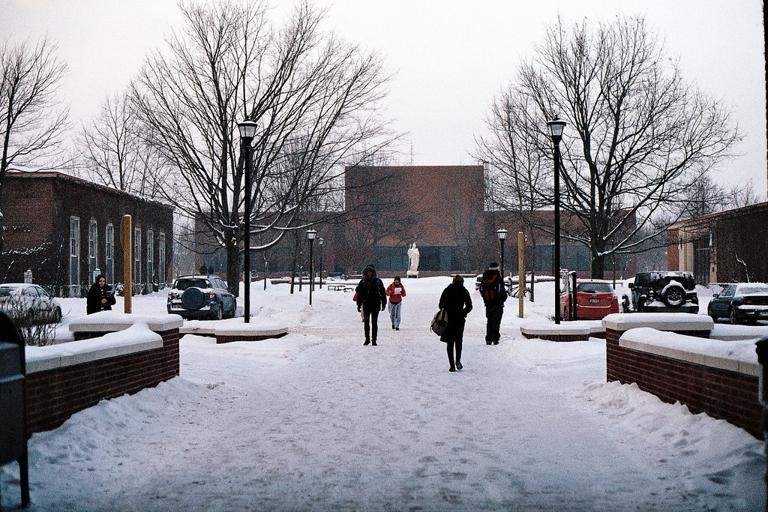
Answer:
[496,226,509,280]
[547,113,567,326]
[306,226,316,306]
[706,282,768,325]
[236,114,259,325]
[317,236,325,288]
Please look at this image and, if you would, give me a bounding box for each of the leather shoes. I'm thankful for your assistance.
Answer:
[357,306,362,312]
[381,306,386,311]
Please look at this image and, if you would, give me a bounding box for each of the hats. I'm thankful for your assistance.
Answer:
[0,282,64,325]
[559,281,620,320]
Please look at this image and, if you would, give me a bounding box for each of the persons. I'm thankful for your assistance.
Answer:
[386,275,406,331]
[355,274,366,322]
[479,262,507,345]
[355,264,387,346]
[438,275,474,372]
[199,263,208,277]
[86,275,117,317]
[207,264,215,276]
[406,241,421,279]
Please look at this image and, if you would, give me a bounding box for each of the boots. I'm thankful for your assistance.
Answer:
[628,270,699,314]
[167,274,237,319]
[475,272,484,291]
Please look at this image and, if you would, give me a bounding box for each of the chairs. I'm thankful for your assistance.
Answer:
[486,339,500,345]
[449,359,464,372]
[363,339,379,346]
[392,325,399,331]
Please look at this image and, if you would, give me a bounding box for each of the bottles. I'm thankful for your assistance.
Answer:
[488,262,499,271]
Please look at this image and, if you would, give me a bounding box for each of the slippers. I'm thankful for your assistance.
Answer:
[481,273,501,304]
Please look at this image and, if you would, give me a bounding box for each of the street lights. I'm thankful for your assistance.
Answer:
[431,307,450,335]
